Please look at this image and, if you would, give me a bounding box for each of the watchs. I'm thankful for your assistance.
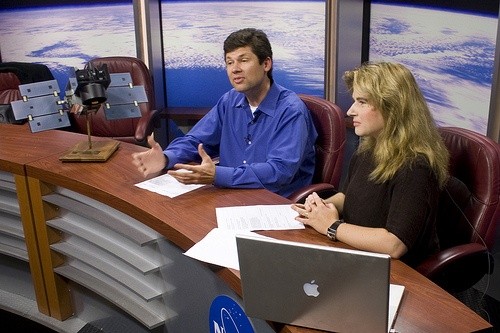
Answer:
[327,218,345,241]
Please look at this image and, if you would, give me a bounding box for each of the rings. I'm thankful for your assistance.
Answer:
[305,211,309,218]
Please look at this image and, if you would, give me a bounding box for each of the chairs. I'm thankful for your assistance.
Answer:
[74,57,152,147]
[0,62,55,124]
[416,127,500,295]
[288,94,345,203]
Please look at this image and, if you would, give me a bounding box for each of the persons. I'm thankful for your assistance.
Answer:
[291,62,450,270]
[132,27,317,204]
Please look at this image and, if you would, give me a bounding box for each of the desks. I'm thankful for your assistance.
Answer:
[0,125,494,333]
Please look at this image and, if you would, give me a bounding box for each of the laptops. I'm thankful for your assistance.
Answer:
[235,235,405,333]
